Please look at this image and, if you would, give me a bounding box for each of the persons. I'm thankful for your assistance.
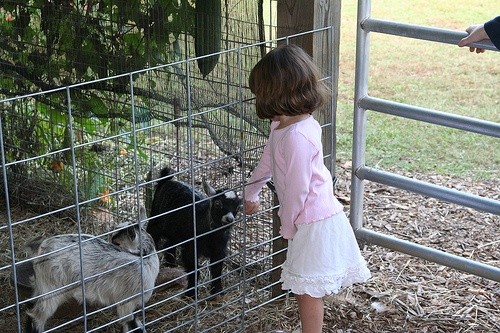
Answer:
[458,16,500,54]
[241,44,372,333]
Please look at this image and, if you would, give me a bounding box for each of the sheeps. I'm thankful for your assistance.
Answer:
[146,166,244,299]
[26,207,160,333]
